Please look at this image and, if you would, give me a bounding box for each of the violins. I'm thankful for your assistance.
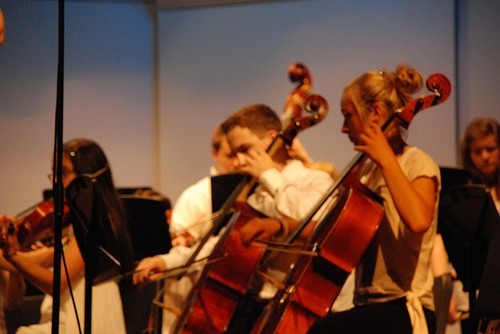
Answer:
[0,201,70,252]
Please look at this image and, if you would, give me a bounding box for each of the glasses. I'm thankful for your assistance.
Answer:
[469,144,498,155]
[49,166,75,182]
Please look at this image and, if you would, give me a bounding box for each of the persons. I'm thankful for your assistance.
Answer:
[440,117,500,334]
[238,65,456,334]
[169,124,338,293]
[0,138,129,334]
[133,103,355,314]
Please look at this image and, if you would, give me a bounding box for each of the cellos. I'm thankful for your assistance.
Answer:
[168,93,329,334]
[278,62,313,135]
[248,73,452,334]
